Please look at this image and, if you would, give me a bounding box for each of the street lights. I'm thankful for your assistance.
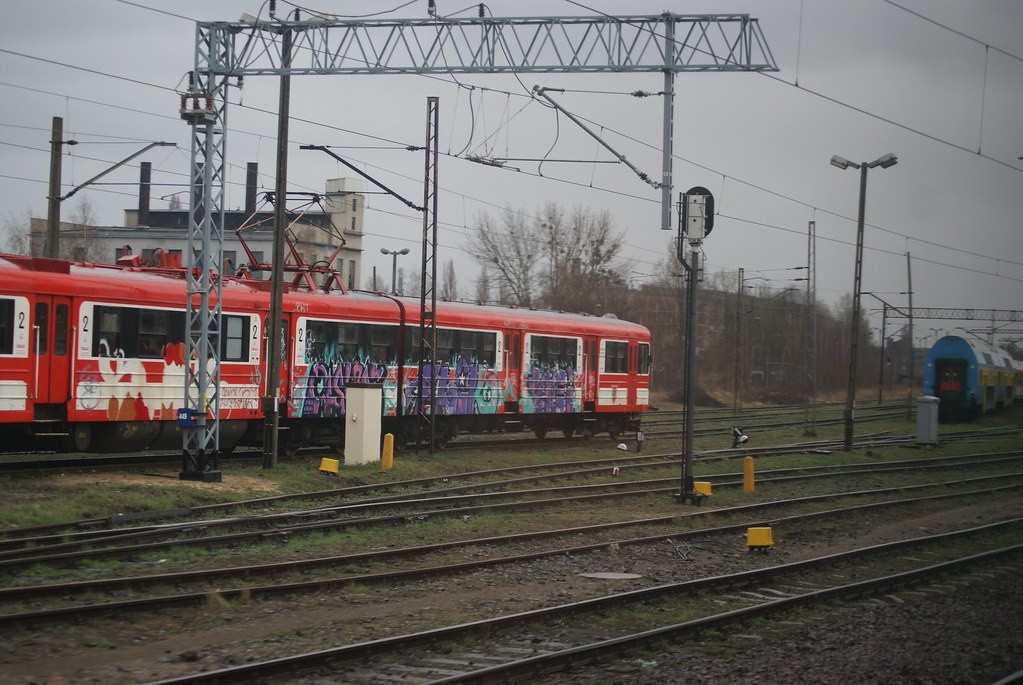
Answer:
[380,247,410,293]
[829,153,899,454]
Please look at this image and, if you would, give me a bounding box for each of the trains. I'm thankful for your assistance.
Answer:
[0,252,659,457]
[923,335,1022,422]
